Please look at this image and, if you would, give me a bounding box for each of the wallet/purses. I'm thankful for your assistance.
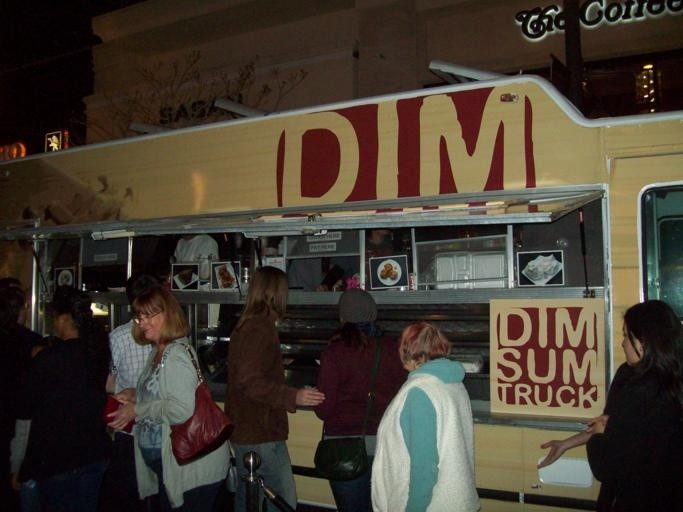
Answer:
[102,395,136,433]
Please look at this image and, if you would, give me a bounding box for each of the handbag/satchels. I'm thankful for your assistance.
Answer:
[314,437,367,481]
[170,381,234,465]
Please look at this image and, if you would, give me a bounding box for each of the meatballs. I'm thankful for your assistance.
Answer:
[379,263,397,280]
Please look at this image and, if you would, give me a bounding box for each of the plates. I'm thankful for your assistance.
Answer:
[522,255,563,286]
[173,272,198,289]
[214,264,239,290]
[376,259,403,287]
[57,269,73,286]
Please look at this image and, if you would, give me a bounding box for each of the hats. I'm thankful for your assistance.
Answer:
[338,288,378,323]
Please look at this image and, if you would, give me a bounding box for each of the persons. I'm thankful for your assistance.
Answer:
[366,321,482,512]
[0,281,44,512]
[224,264,326,511]
[584,297,683,511]
[103,287,235,512]
[536,362,633,470]
[310,286,408,512]
[7,283,111,510]
[162,233,219,289]
[366,230,394,265]
[103,275,162,511]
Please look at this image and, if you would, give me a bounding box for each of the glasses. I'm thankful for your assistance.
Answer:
[134,311,161,325]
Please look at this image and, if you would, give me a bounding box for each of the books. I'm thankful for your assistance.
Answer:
[99,393,134,433]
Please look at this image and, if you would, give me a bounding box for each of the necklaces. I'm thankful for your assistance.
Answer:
[151,355,160,365]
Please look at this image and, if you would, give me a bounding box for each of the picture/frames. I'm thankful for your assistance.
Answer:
[368,254,411,290]
[170,260,242,291]
[516,249,566,288]
[52,267,78,293]
